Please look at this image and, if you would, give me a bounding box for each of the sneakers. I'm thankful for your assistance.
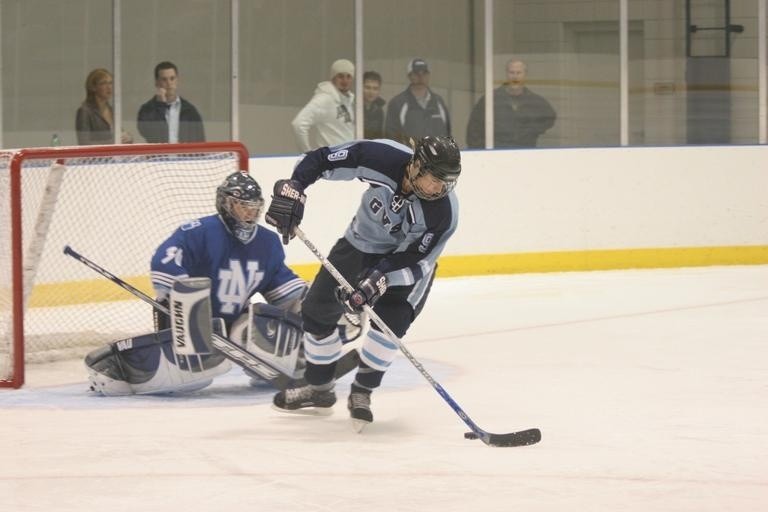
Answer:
[348,390,372,421]
[274,386,335,409]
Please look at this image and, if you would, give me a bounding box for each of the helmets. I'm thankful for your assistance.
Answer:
[216,172,265,241]
[408,136,461,201]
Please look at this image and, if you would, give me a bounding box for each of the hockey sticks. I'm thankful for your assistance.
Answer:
[292,225,541,447]
[64,246,362,390]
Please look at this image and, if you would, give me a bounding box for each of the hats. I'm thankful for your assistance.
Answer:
[407,57,431,75]
[331,59,355,80]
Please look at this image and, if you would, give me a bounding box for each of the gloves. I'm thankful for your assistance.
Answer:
[266,179,306,245]
[333,267,388,314]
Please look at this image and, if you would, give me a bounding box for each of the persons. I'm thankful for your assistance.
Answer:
[267,135,461,422]
[73,68,133,142]
[388,58,452,149]
[363,72,386,138]
[293,59,355,152]
[84,170,362,393]
[467,61,557,147]
[138,62,206,144]
